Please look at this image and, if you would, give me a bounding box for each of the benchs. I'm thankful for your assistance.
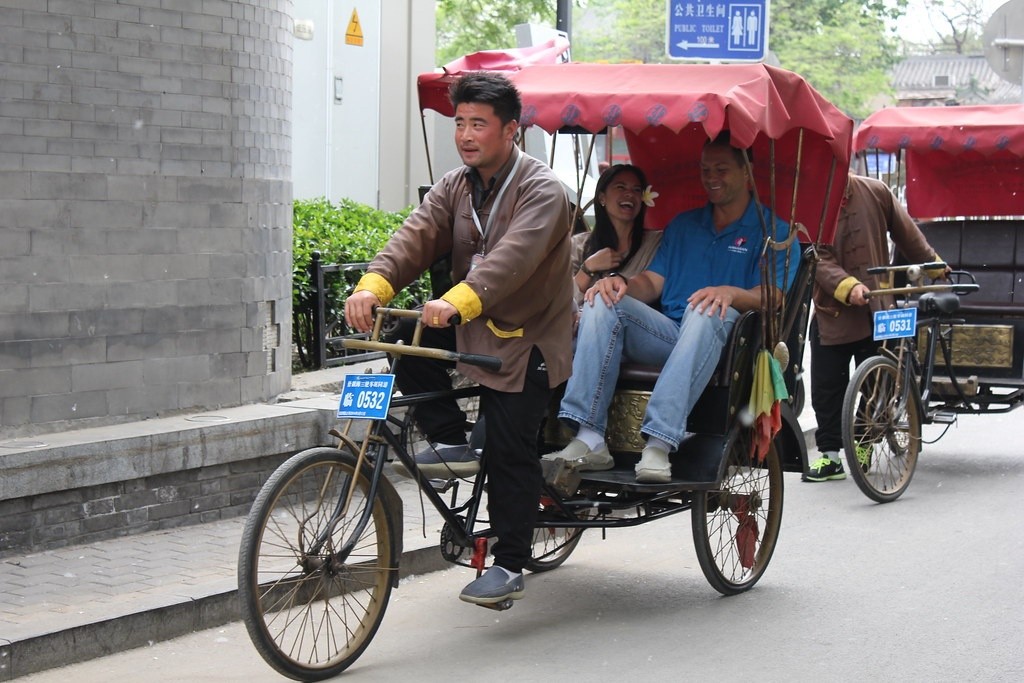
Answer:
[889,215,1024,319]
[538,240,813,391]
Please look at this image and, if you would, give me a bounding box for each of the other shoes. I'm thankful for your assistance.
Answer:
[634,446,672,482]
[458,566,525,603]
[392,443,481,479]
[542,437,615,472]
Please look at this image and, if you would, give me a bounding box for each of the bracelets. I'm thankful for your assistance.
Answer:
[581,262,595,277]
[609,273,628,286]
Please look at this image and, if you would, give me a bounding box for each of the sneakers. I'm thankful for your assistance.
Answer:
[802,454,847,481]
[854,442,874,475]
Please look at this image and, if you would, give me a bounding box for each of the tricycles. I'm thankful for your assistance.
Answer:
[234,63,856,683]
[840,100,1023,506]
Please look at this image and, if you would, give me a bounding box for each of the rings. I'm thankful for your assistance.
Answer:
[714,299,721,302]
[432,316,438,324]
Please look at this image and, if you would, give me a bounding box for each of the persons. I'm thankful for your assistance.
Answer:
[345,70,574,603]
[801,174,952,483]
[541,129,801,482]
